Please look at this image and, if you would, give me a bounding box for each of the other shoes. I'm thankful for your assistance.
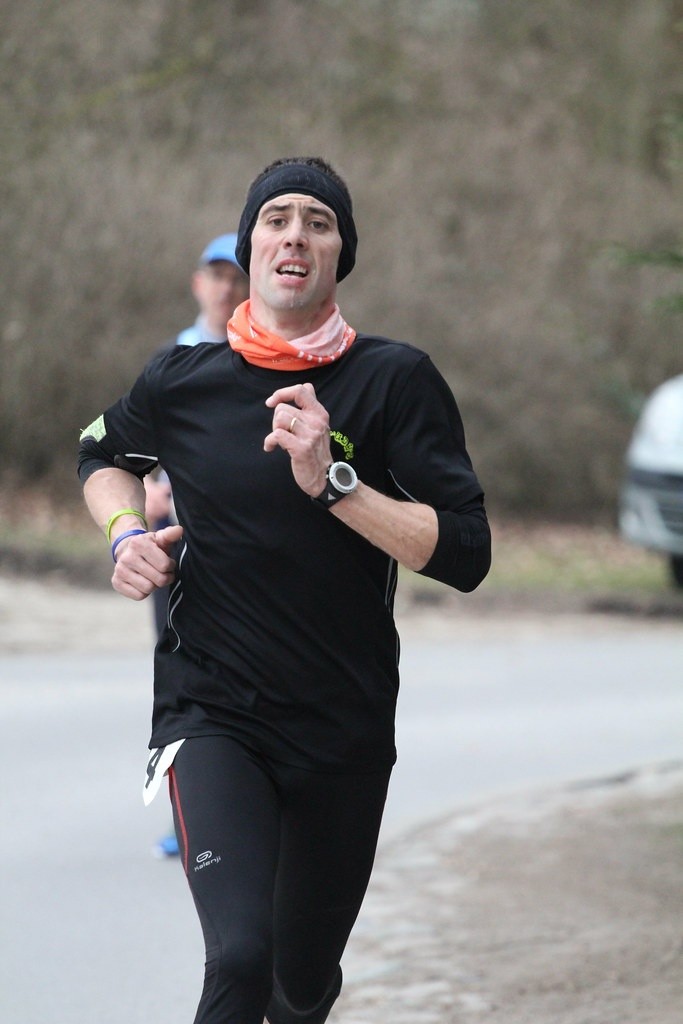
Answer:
[158,827,179,855]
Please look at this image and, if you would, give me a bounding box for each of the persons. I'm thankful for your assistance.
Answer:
[145,233,250,855]
[74,157,492,1024]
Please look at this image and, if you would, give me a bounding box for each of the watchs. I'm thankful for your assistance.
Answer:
[313,461,359,509]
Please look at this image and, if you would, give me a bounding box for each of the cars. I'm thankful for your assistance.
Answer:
[617,371,683,593]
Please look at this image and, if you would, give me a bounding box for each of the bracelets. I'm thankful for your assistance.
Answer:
[111,529,146,563]
[106,508,148,543]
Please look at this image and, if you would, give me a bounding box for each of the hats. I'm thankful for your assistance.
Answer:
[200,231,245,273]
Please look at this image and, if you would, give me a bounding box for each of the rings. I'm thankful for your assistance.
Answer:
[290,417,297,432]
